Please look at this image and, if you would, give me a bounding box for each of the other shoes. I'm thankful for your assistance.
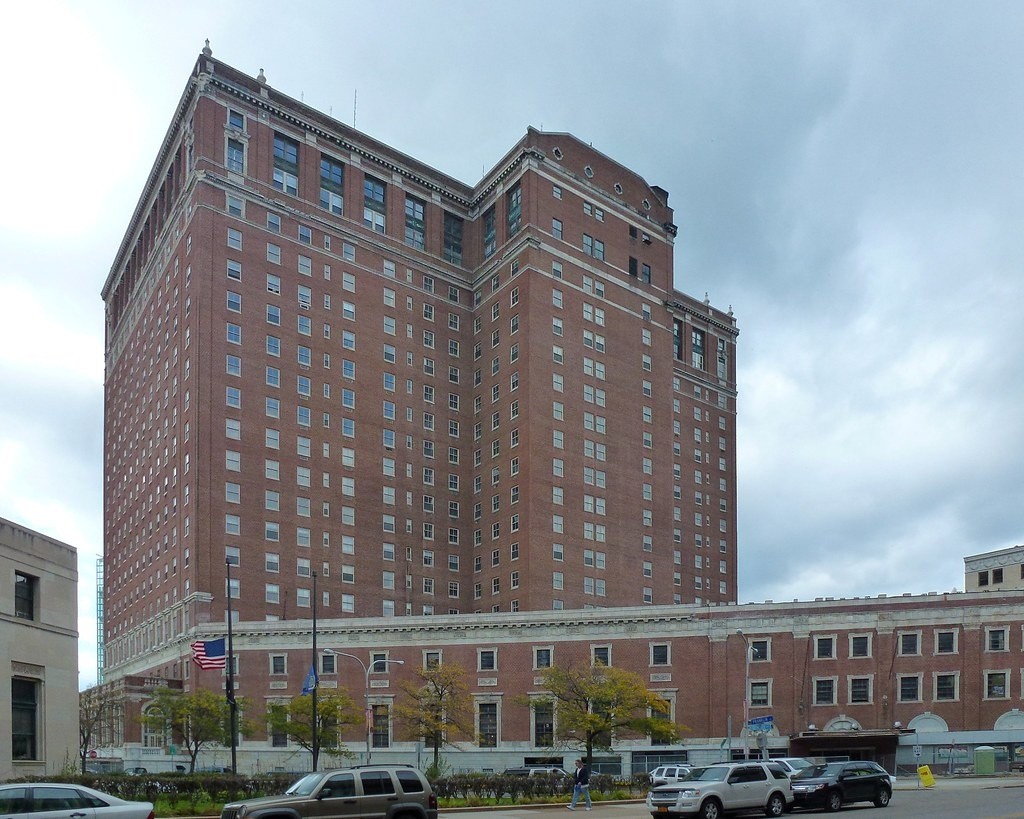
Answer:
[585,807,592,811]
[567,806,575,811]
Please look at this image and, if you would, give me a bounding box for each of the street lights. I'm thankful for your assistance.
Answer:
[323,648,404,766]
[736,628,759,761]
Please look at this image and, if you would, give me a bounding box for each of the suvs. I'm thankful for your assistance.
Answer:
[647,760,794,819]
[652,764,694,788]
[220,764,438,819]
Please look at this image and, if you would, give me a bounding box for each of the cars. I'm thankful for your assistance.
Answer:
[783,761,893,812]
[763,758,816,783]
[0,782,154,819]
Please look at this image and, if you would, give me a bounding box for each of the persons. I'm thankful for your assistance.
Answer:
[566,759,593,811]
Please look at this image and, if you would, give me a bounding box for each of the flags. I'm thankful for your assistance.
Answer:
[190,638,226,670]
[300,663,315,696]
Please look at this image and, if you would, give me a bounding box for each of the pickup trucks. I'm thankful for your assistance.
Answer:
[501,768,572,793]
[124,767,160,775]
